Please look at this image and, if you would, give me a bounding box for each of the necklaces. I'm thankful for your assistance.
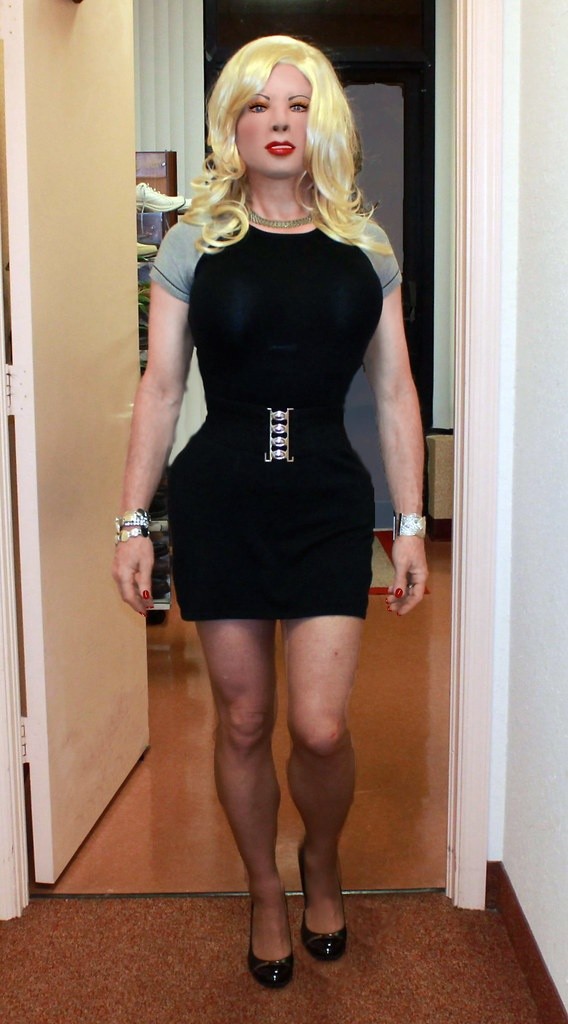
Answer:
[243,202,316,230]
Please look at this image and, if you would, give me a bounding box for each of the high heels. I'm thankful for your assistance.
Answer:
[248,893,293,990]
[298,843,345,961]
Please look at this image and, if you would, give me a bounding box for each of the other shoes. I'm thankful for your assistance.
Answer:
[144,474,171,598]
[137,241,159,261]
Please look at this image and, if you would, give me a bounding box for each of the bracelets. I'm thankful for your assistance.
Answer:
[113,507,152,545]
[390,510,427,541]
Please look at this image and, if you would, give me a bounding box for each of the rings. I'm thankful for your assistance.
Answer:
[407,583,415,588]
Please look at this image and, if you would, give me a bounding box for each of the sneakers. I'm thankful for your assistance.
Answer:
[136,181,186,235]
[179,196,193,213]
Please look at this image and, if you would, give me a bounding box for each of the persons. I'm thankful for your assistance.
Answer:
[110,28,428,988]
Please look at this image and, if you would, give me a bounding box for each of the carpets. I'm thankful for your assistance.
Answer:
[369,531,431,596]
